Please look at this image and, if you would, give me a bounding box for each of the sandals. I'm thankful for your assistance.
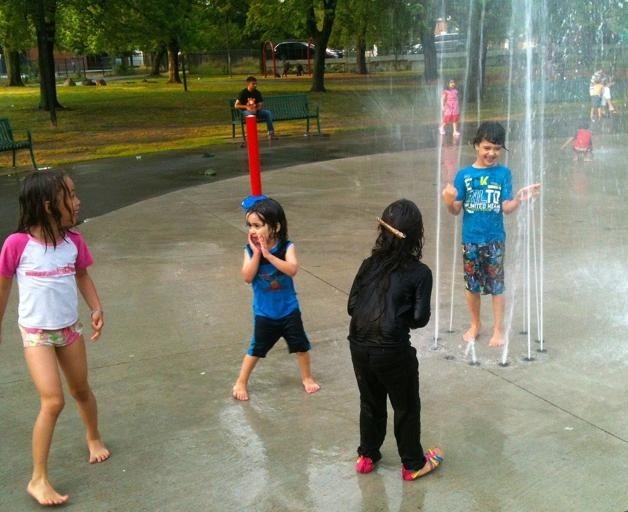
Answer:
[355,456,376,472]
[402,449,444,480]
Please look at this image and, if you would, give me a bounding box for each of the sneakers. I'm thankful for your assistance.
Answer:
[437,127,446,135]
[268,134,277,140]
[452,131,461,136]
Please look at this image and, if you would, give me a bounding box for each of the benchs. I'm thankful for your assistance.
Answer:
[228,90,321,143]
[0,113,41,178]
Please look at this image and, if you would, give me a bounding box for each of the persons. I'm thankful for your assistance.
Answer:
[439,78,461,137]
[441,119,548,351]
[0,167,112,507]
[233,76,280,141]
[559,119,595,163]
[588,62,617,124]
[228,198,323,406]
[344,199,445,486]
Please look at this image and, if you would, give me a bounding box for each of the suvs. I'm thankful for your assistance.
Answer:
[407,31,468,56]
[271,41,343,59]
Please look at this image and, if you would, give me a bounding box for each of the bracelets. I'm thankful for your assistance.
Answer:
[89,310,104,318]
